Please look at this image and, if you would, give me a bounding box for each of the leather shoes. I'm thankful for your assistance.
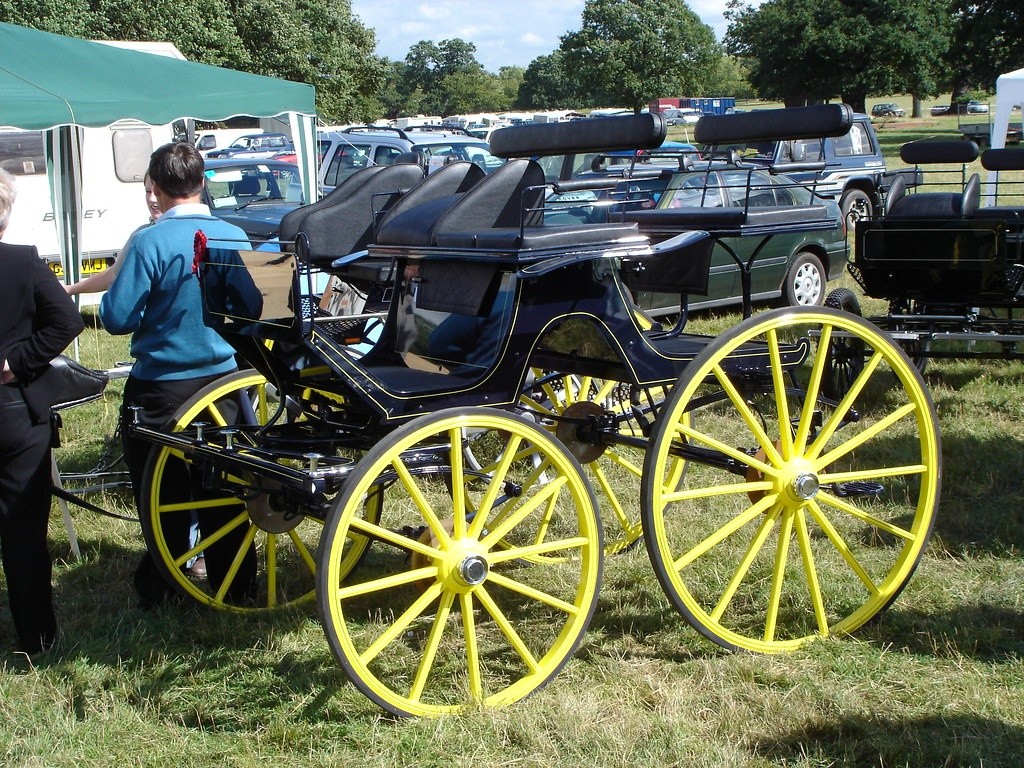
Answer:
[189,556,208,579]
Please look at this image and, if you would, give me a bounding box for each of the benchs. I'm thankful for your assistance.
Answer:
[278,104,1024,297]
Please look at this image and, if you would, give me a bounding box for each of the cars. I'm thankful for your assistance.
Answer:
[930,104,949,116]
[967,100,989,114]
[177,107,887,329]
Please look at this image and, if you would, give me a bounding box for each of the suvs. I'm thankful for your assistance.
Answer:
[872,102,907,119]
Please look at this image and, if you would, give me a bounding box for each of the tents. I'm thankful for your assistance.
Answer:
[0,21,316,363]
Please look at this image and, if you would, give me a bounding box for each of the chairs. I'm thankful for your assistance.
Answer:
[231,176,261,199]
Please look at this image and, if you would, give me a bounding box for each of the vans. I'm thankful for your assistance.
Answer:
[0,40,193,312]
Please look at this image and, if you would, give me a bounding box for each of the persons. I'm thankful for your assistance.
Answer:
[0,167,85,661]
[64,170,206,578]
[99,142,253,605]
